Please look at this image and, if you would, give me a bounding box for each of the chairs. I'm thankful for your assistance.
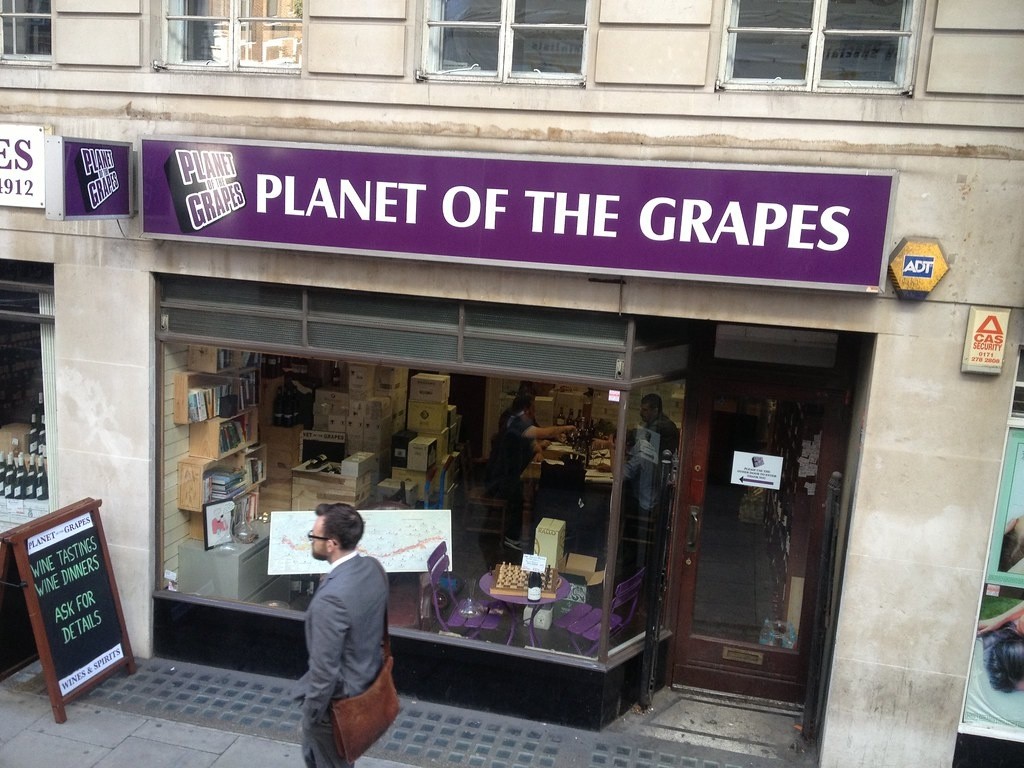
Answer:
[554,566,649,657]
[490,433,500,483]
[426,541,505,639]
[458,439,511,549]
[617,461,671,565]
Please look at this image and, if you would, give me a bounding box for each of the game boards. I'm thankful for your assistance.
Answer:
[489,561,560,599]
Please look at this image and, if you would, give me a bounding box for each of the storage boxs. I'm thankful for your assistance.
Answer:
[559,553,603,614]
[502,382,684,430]
[523,604,554,630]
[291,361,461,522]
[534,518,565,568]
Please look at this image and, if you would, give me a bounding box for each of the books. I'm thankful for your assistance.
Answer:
[217,348,237,370]
[244,457,264,486]
[238,351,259,368]
[237,374,257,412]
[218,412,253,455]
[232,491,260,530]
[200,465,249,505]
[187,383,234,423]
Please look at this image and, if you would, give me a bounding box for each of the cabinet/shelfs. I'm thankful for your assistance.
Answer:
[173,344,267,542]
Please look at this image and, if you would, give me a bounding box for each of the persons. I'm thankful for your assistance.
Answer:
[483,398,577,551]
[590,393,680,461]
[496,380,552,450]
[590,428,674,571]
[287,503,391,768]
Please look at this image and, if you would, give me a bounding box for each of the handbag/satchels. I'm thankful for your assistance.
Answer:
[330,656,401,766]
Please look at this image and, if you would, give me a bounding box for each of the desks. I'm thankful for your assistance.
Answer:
[478,568,571,649]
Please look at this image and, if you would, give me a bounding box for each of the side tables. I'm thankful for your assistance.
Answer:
[259,424,303,478]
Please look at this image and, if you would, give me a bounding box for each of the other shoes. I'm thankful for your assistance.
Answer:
[504,535,522,550]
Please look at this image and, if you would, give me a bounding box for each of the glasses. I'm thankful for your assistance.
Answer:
[308,530,337,546]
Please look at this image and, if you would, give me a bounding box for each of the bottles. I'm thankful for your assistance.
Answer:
[273,391,284,425]
[575,409,581,428]
[284,390,292,427]
[293,389,300,426]
[214,516,242,556]
[332,360,341,388]
[306,454,328,470]
[529,554,542,602]
[458,578,484,618]
[235,497,256,542]
[261,353,311,381]
[567,408,574,425]
[556,407,565,426]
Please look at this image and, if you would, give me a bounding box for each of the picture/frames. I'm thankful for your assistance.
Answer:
[202,497,234,551]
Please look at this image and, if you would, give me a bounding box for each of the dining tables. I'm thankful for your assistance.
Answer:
[518,438,615,566]
